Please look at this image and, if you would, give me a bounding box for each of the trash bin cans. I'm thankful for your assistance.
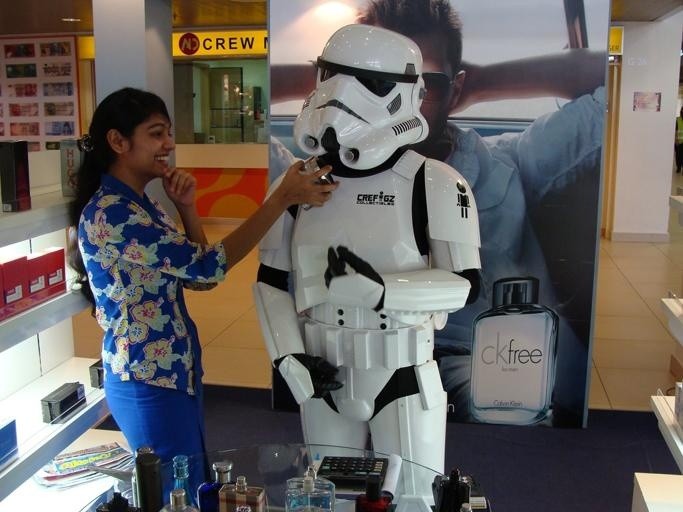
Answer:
[208,136,215,144]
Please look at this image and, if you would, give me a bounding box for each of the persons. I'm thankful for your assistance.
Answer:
[268,0,606,428]
[251,23,486,511]
[64,87,340,508]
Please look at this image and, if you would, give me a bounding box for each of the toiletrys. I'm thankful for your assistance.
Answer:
[297,157,335,211]
[468,276,558,425]
[129,446,335,512]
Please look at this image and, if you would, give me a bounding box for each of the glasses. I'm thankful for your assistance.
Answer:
[422,71,456,101]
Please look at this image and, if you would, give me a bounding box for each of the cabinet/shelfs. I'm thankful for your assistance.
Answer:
[0,215,136,512]
[628,193,683,511]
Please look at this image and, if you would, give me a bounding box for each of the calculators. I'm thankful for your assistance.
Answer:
[317,456,388,489]
